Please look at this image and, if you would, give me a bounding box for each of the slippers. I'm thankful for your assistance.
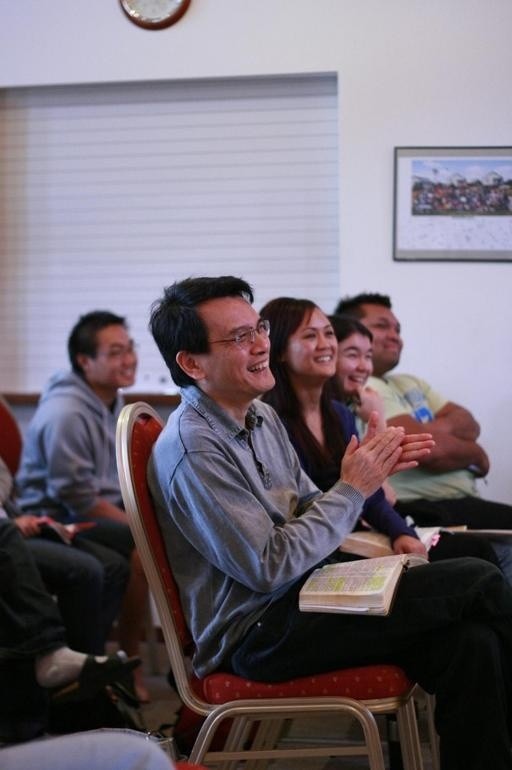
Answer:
[52,652,142,702]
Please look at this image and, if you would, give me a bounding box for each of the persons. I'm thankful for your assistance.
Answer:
[1,456,145,735]
[322,315,398,509]
[147,274,512,770]
[258,296,512,579]
[9,308,182,693]
[331,288,512,584]
[0,516,144,749]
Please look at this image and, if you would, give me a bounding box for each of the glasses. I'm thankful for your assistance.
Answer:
[209,320,270,349]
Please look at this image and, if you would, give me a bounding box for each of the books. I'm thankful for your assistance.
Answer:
[34,515,99,548]
[340,525,455,560]
[298,553,429,617]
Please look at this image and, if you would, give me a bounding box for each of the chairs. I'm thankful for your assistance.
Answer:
[1,392,439,769]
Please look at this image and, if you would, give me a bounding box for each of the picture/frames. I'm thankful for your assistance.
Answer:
[389,142,510,265]
